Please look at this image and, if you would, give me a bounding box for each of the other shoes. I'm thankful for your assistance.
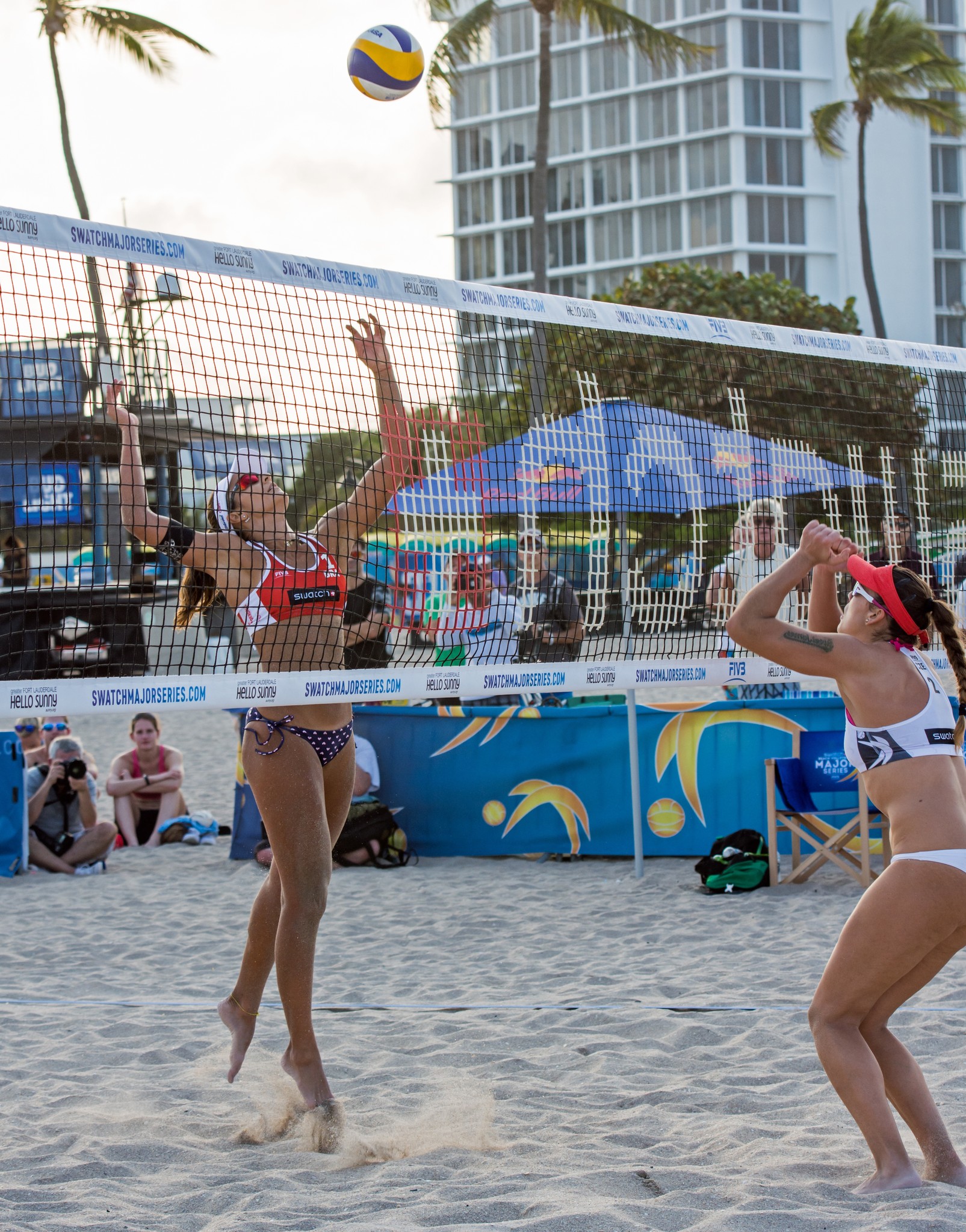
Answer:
[182,827,201,845]
[74,860,106,876]
[200,835,216,845]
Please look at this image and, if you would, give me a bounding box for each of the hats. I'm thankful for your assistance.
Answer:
[214,447,268,536]
[747,498,782,520]
[518,529,545,545]
[445,549,465,568]
[884,507,912,522]
[848,555,930,644]
[459,563,482,577]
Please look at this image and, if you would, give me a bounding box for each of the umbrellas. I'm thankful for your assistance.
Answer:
[381,398,891,879]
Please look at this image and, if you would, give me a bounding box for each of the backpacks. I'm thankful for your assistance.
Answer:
[333,802,398,856]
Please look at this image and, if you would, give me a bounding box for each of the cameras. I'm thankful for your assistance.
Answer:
[59,757,86,782]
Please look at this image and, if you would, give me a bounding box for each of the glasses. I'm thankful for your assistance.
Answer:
[886,521,909,528]
[15,725,35,733]
[754,518,773,526]
[350,551,362,558]
[231,473,258,503]
[521,543,541,550]
[41,723,67,732]
[852,581,892,617]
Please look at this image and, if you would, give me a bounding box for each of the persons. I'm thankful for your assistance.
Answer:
[15,716,118,875]
[422,527,586,705]
[852,508,939,652]
[724,519,966,1193]
[704,498,800,698]
[102,713,191,856]
[343,537,392,708]
[254,733,398,866]
[106,313,413,1128]
[0,535,30,587]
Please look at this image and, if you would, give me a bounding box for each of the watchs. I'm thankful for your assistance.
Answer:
[143,773,150,786]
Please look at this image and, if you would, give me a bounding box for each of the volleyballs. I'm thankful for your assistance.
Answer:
[346,24,426,102]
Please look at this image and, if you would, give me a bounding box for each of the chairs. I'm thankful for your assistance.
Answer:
[765,729,892,890]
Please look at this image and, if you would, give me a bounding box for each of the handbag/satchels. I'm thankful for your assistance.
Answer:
[695,828,770,893]
